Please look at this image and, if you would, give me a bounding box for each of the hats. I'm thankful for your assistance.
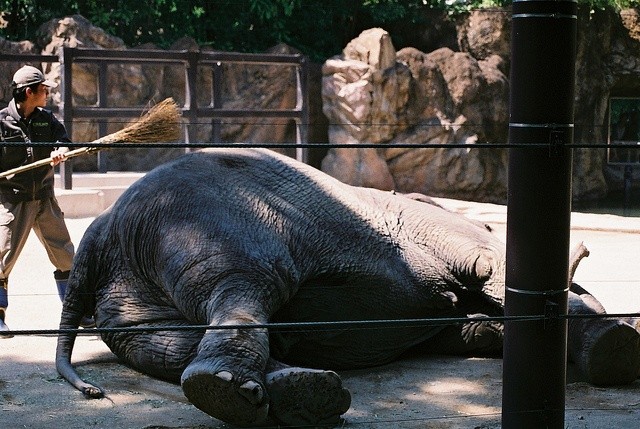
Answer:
[13,65,59,90]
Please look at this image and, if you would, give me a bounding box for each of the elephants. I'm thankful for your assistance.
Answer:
[55,147,640,429]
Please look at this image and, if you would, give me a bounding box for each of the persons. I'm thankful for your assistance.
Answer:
[0,65,96,340]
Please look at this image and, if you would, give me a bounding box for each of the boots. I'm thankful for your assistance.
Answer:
[54,269,95,330]
[0,279,14,339]
